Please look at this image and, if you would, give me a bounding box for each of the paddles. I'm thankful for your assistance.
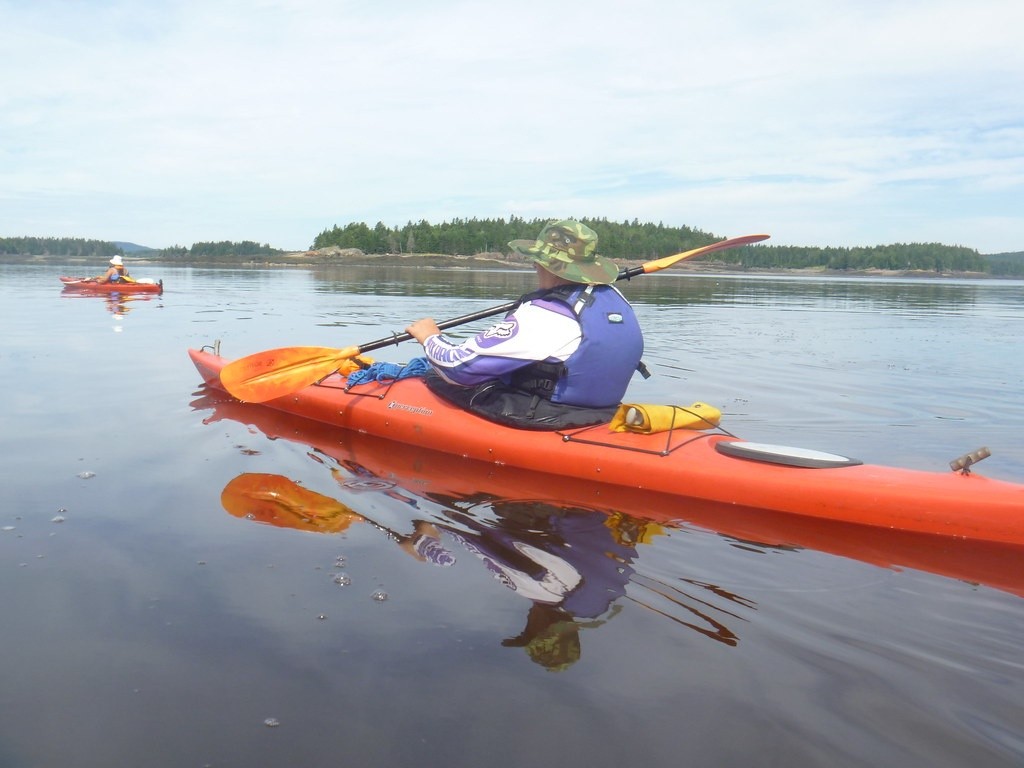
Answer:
[63,276,97,286]
[220,472,403,543]
[218,233,771,405]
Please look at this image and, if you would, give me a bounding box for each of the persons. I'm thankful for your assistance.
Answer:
[398,500,644,673]
[93,254,130,285]
[404,218,645,432]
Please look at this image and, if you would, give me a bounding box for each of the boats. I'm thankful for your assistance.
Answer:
[197,383,1024,596]
[190,347,1024,547]
[60,274,163,294]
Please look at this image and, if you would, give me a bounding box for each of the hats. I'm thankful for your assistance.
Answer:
[502,605,606,672]
[507,219,619,284]
[110,255,123,265]
